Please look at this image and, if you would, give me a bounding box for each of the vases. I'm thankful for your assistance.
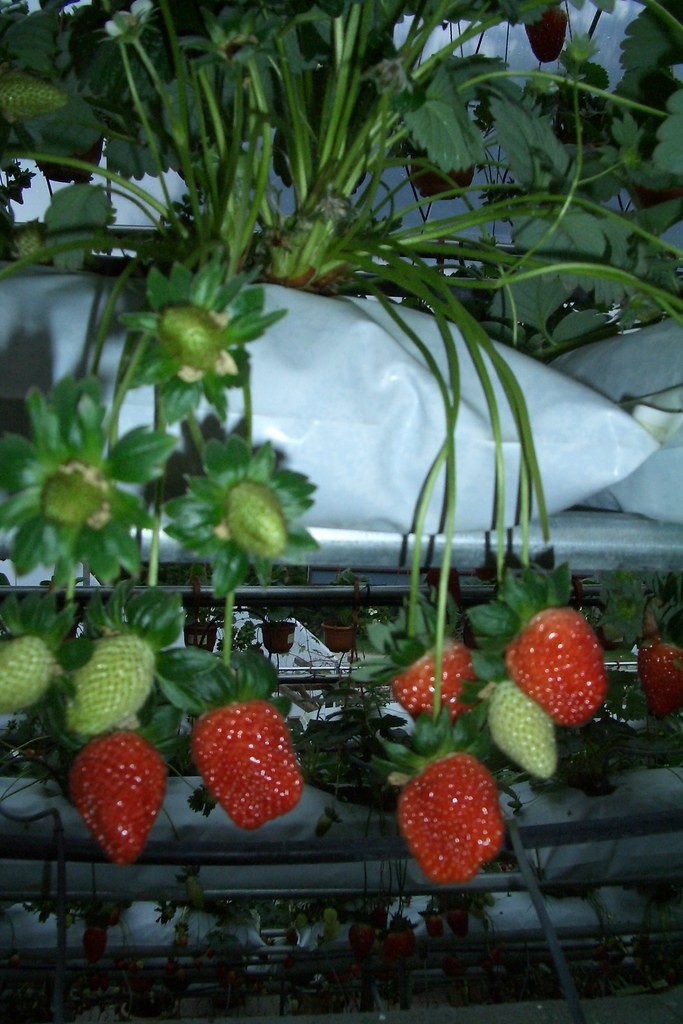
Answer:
[258,622,297,654]
[184,620,217,653]
[120,997,184,1020]
[321,619,357,652]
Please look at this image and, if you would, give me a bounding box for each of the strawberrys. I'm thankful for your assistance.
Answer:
[0,303,683,887]
